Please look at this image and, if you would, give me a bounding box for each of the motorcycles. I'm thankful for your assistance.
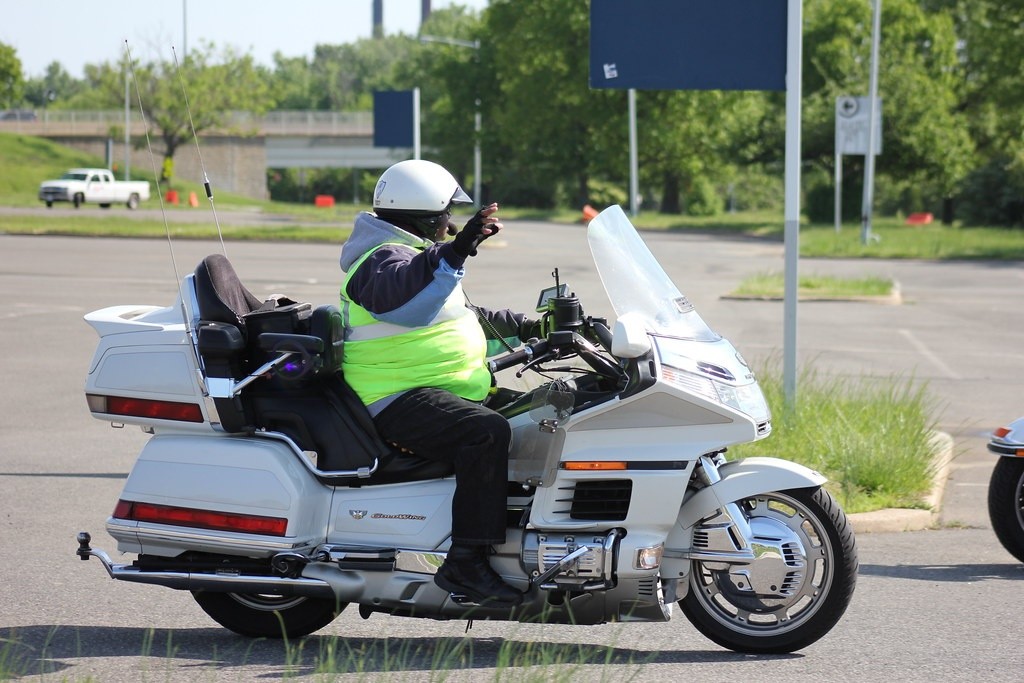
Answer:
[77,204,858,653]
[987,415,1024,563]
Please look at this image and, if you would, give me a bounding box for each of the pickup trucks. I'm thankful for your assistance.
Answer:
[38,169,150,208]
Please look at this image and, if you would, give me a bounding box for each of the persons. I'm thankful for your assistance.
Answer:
[337,159,544,610]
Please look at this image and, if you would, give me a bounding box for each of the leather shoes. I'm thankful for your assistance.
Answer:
[435,539,524,609]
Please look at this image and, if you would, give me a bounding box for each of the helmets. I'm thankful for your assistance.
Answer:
[374,159,460,216]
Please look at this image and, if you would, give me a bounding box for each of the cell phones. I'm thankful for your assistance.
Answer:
[535,282,570,312]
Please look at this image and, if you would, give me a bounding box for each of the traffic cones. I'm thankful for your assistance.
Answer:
[188,192,199,207]
